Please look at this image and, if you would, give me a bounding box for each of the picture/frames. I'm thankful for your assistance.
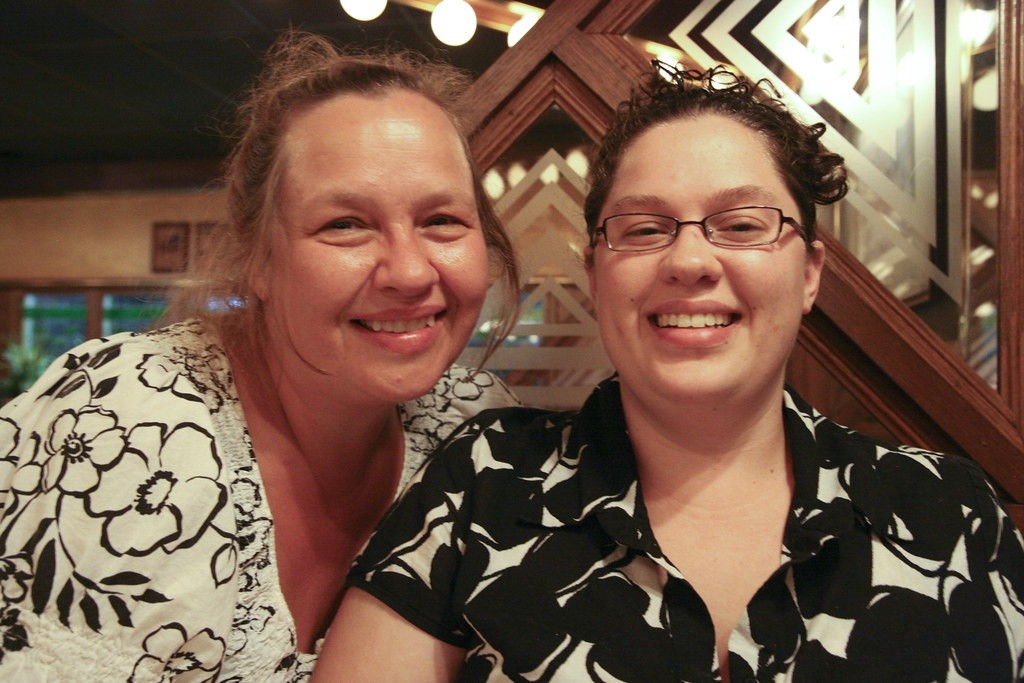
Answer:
[150,221,190,272]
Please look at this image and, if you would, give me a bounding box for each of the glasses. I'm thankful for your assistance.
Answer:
[590,204,810,249]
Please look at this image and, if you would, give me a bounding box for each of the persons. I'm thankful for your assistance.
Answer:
[309,60,1024,683]
[0,32,522,683]
